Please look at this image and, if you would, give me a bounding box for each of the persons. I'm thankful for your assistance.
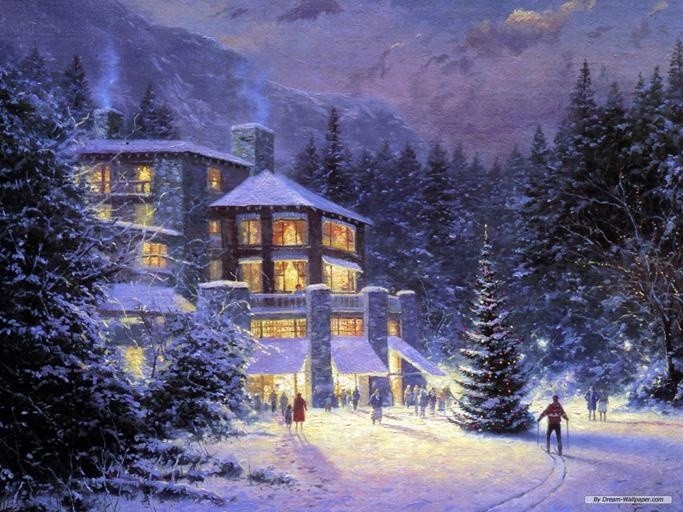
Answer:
[586,386,601,423]
[254,387,263,417]
[368,388,383,426]
[353,385,361,411]
[293,284,305,294]
[341,388,348,410]
[269,390,280,413]
[596,387,609,424]
[294,393,310,434]
[324,395,333,413]
[284,404,294,434]
[280,392,289,418]
[403,384,451,421]
[537,395,570,457]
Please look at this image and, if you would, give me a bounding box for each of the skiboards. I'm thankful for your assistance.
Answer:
[540,444,565,462]
